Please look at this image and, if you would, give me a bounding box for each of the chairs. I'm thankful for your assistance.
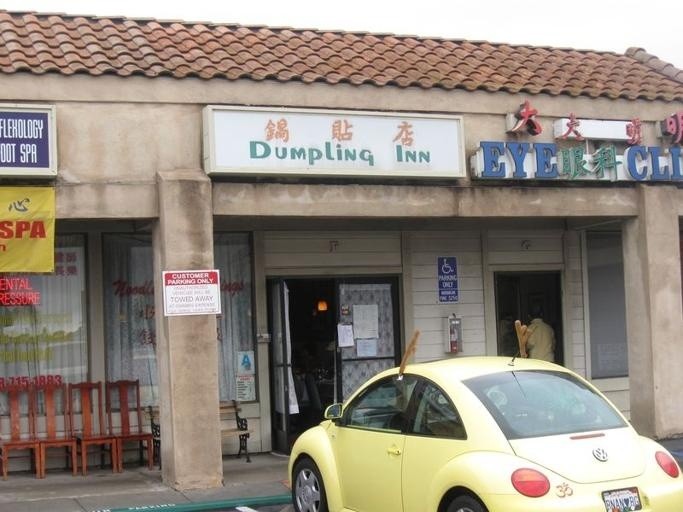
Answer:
[1,380,153,479]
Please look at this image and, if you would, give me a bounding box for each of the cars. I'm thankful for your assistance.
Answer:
[288,356,683,512]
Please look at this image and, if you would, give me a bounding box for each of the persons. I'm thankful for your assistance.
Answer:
[269,328,302,432]
[523,305,556,363]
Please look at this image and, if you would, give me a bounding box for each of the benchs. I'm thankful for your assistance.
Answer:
[145,400,253,470]
